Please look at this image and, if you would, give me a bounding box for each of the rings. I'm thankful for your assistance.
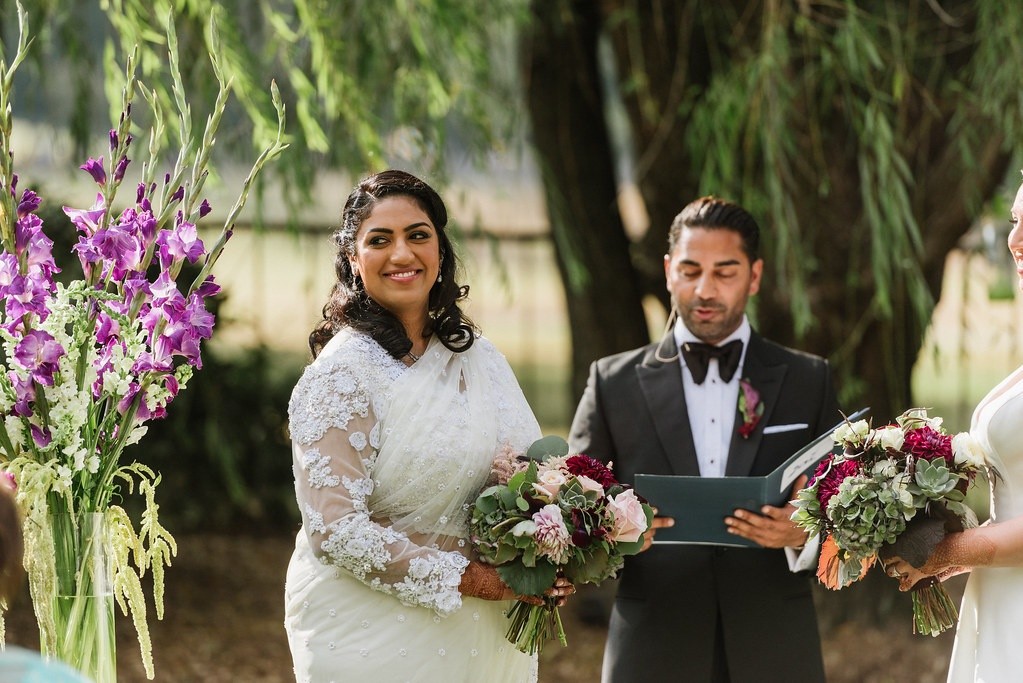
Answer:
[891,565,901,579]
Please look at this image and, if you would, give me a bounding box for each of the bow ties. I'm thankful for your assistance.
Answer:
[680,339,744,384]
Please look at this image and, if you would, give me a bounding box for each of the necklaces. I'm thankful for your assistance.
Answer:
[405,350,423,363]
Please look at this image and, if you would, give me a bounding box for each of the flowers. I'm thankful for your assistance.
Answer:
[0,0,292,682]
[467,435,657,658]
[790,406,1007,641]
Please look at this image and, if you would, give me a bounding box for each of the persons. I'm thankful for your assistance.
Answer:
[565,195,850,683]
[285,169,546,683]
[874,169,1023,683]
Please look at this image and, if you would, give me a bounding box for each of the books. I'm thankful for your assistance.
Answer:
[633,406,876,551]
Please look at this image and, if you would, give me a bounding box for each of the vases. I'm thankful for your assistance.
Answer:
[22,512,118,683]
